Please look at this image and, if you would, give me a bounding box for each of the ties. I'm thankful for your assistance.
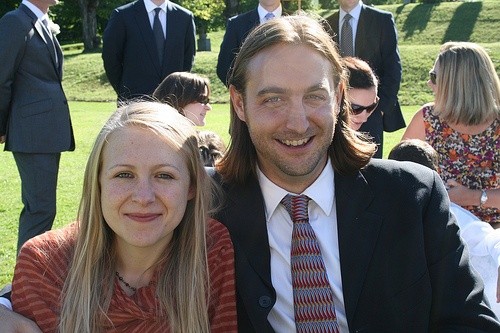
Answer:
[38,20,59,69]
[279,196,342,333]
[152,8,167,66]
[265,12,274,22]
[340,12,354,56]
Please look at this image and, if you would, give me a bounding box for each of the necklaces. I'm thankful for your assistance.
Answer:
[114,272,136,293]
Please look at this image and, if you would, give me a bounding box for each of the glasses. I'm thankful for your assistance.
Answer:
[196,94,211,104]
[348,102,378,116]
[426,71,437,85]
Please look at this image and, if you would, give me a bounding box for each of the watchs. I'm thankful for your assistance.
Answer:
[480,188,489,210]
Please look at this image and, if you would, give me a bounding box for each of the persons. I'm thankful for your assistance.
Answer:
[318,0,407,159]
[102,0,198,111]
[152,71,228,168]
[401,41,500,230]
[10,101,238,333]
[0,0,76,259]
[216,0,298,105]
[0,16,500,333]
[337,56,500,322]
